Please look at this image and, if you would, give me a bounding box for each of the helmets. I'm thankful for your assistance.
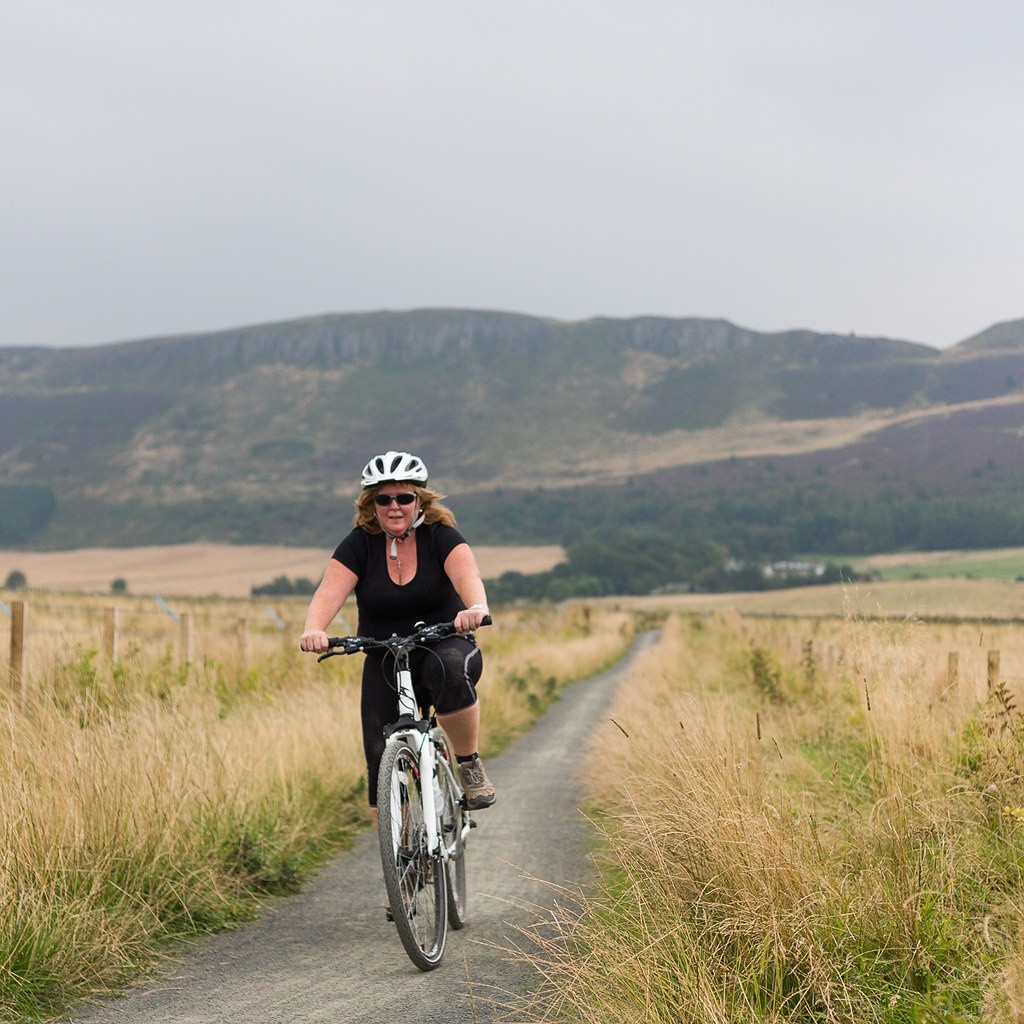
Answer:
[361,450,428,491]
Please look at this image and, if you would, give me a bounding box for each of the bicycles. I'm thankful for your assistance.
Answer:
[316,615,490,972]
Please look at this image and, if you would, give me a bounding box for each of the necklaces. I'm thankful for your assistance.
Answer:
[396,558,402,569]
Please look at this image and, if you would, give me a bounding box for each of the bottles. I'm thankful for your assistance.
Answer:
[431,770,445,817]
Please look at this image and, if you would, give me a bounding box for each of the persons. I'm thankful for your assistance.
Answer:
[298,450,498,922]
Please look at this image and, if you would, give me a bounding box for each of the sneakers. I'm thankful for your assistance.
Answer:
[384,866,417,922]
[457,758,498,810]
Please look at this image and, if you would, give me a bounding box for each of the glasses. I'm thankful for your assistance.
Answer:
[371,491,417,507]
